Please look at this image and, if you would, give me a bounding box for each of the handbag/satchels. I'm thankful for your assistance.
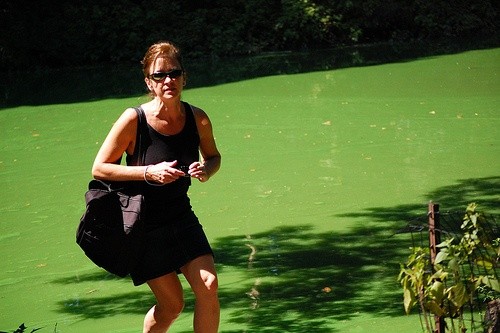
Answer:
[69,178,163,276]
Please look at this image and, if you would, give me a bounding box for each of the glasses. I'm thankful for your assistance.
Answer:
[146,66,185,81]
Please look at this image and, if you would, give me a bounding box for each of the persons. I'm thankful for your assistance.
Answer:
[92,41,222,333]
